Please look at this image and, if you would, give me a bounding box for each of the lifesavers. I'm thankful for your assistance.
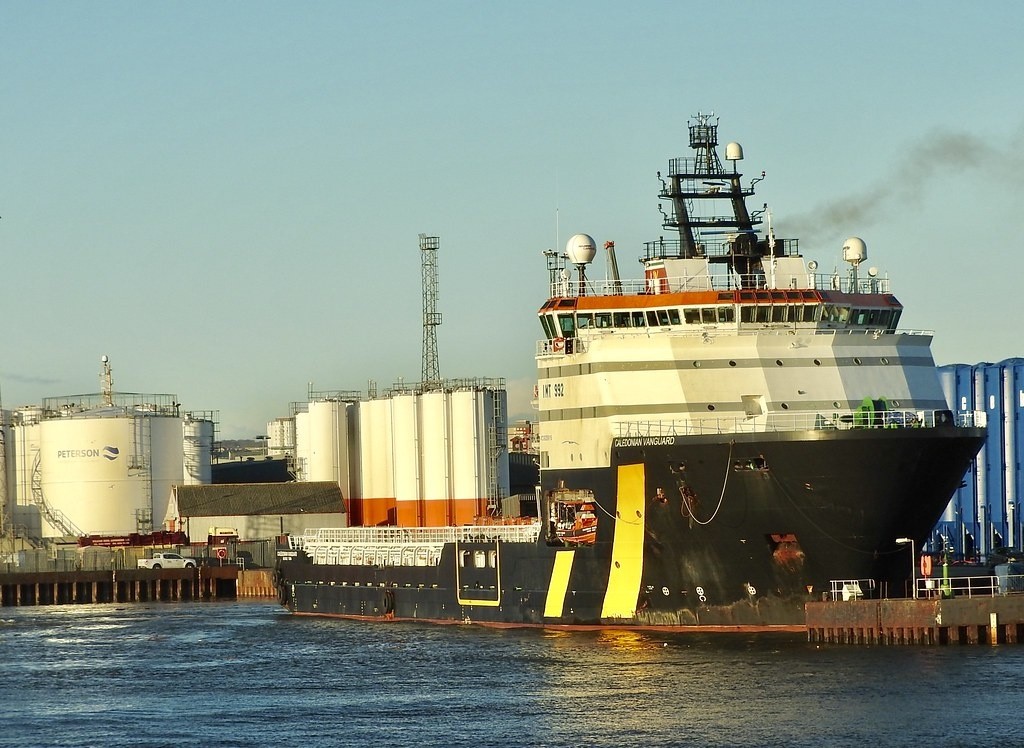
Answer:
[554,337,565,349]
[218,550,226,558]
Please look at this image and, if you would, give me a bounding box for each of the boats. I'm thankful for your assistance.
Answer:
[555,504,598,544]
[272,113,988,633]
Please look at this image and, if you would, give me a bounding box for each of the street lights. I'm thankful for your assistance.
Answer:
[895,539,916,600]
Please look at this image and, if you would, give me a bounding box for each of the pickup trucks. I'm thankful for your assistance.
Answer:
[137,552,197,569]
